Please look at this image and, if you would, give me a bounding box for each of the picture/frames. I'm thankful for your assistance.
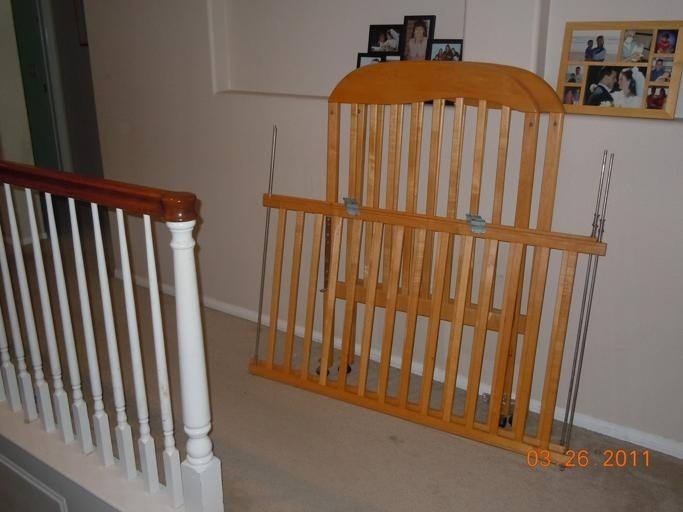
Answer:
[553,19,682,120]
[357,14,463,64]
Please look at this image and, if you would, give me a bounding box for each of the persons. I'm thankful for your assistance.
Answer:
[379,28,397,50]
[441,45,452,60]
[404,19,427,59]
[446,48,460,62]
[433,48,443,62]
[375,32,385,48]
[563,28,672,113]
